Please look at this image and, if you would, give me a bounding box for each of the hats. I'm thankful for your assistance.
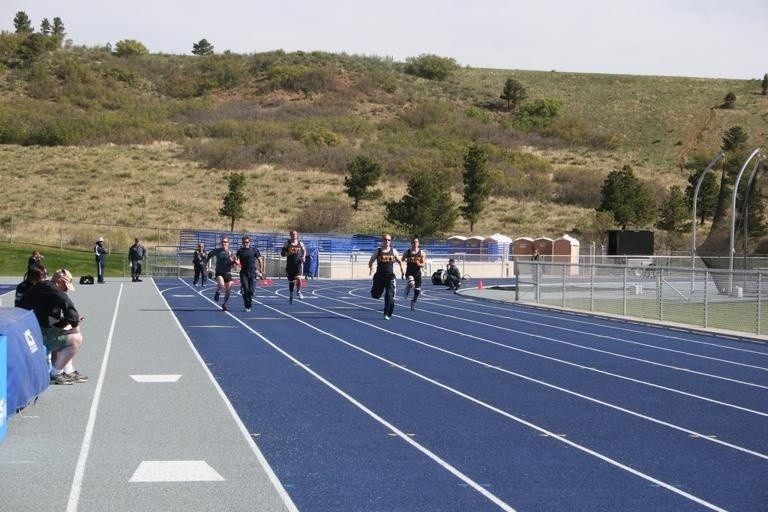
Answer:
[54,269,75,292]
[97,237,104,241]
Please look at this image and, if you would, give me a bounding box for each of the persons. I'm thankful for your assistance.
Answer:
[401,234,426,311]
[235,236,263,312]
[192,243,208,287]
[368,234,405,320]
[128,237,148,282]
[446,258,460,290]
[203,237,237,311]
[531,248,543,284]
[94,236,108,284]
[281,230,307,305]
[15,250,89,386]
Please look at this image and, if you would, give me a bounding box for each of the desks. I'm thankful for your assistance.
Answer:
[1,305,51,440]
[1,335,7,444]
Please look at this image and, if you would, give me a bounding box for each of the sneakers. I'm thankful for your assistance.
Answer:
[222,304,228,310]
[296,291,304,299]
[383,315,390,320]
[215,291,220,301]
[49,370,88,385]
[411,300,416,310]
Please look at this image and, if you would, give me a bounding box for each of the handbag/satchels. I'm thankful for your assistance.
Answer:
[80,273,94,284]
